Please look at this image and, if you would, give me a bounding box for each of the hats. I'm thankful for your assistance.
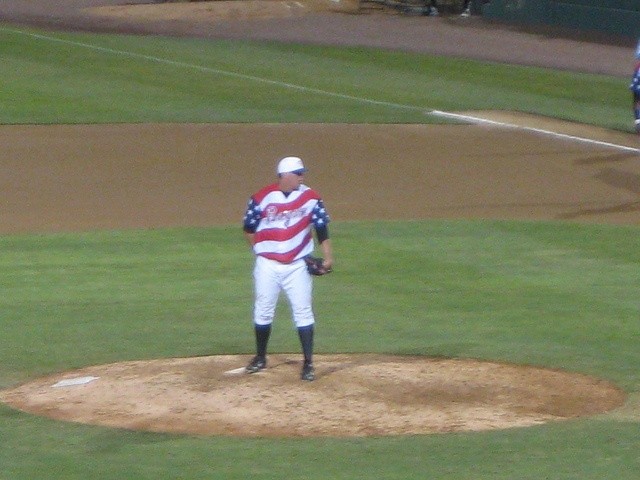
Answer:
[277,157,309,174]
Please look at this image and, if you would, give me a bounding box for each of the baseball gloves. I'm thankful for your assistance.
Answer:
[303,254,332,276]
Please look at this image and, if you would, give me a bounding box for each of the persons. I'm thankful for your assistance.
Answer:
[629,42,640,135]
[245,156,334,380]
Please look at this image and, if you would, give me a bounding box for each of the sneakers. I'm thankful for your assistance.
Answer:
[302,360,314,380]
[246,356,265,371]
